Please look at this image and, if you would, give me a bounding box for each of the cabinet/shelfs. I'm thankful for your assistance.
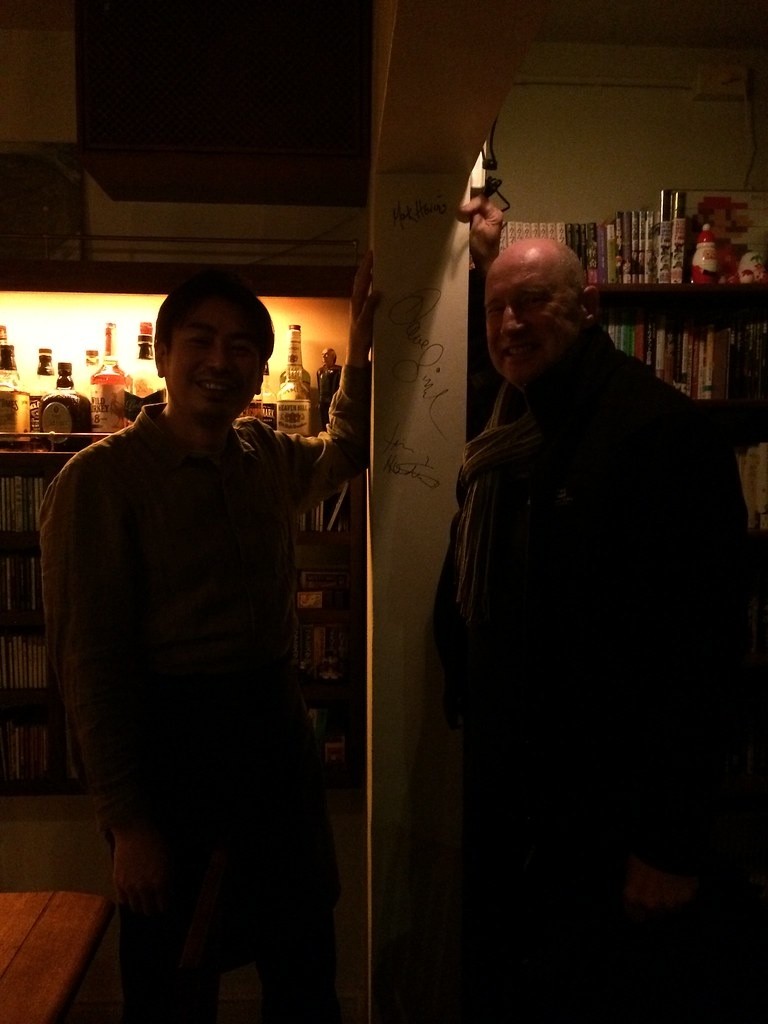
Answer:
[486,184,768,553]
[0,289,356,794]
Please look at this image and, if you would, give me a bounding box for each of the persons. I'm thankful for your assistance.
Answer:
[38,242,380,1021]
[432,192,768,1024]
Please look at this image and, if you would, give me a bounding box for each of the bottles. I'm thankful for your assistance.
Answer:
[0,320,314,453]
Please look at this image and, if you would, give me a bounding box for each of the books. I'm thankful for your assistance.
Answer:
[495,188,687,285]
[597,290,767,400]
[709,575,768,902]
[287,482,353,768]
[1,474,51,778]
[729,435,768,532]
